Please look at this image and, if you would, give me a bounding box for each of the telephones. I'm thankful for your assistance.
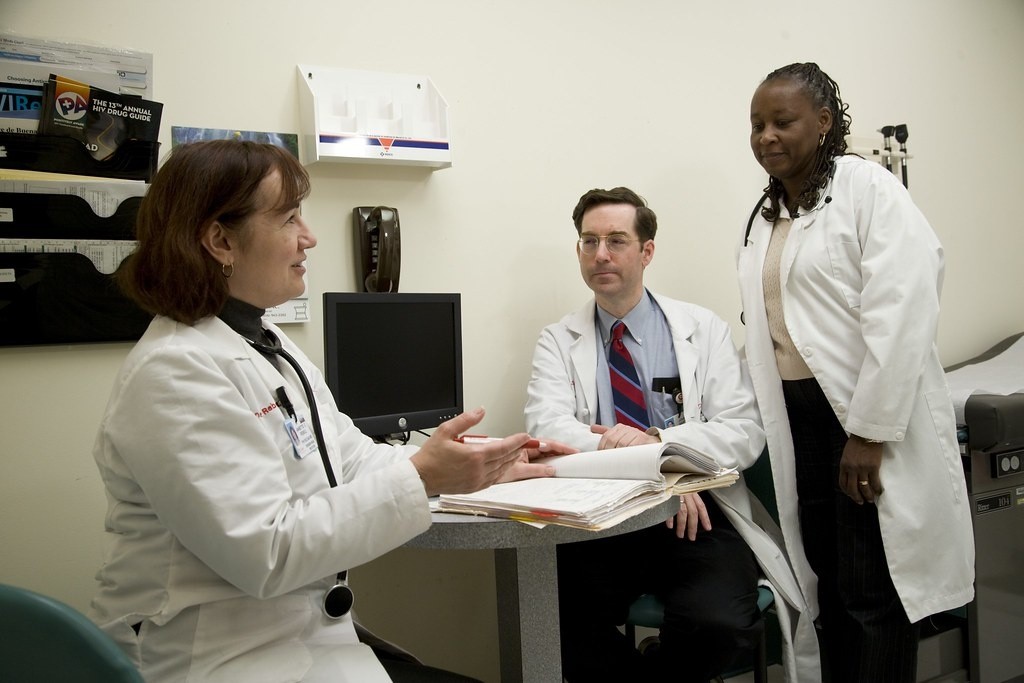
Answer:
[352,204,403,293]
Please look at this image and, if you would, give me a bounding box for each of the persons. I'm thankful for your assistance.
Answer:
[524,186,766,683]
[738,63,975,683]
[93,141,580,683]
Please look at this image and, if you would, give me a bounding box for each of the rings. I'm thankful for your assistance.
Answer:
[680,500,686,503]
[858,480,869,485]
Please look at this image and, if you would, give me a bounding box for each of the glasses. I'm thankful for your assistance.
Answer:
[579,234,641,255]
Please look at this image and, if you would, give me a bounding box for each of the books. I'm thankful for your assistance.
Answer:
[428,441,739,531]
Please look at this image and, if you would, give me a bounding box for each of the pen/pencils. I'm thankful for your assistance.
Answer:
[660,386,666,408]
[275,385,299,424]
[453,433,548,449]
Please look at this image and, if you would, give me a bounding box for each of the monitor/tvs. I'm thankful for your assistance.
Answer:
[323,292,463,445]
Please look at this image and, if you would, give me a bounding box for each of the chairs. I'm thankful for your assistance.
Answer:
[0,582,147,683]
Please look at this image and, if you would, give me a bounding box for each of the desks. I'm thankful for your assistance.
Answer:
[401,495,680,683]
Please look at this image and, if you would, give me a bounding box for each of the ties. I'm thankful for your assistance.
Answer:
[609,320,651,432]
